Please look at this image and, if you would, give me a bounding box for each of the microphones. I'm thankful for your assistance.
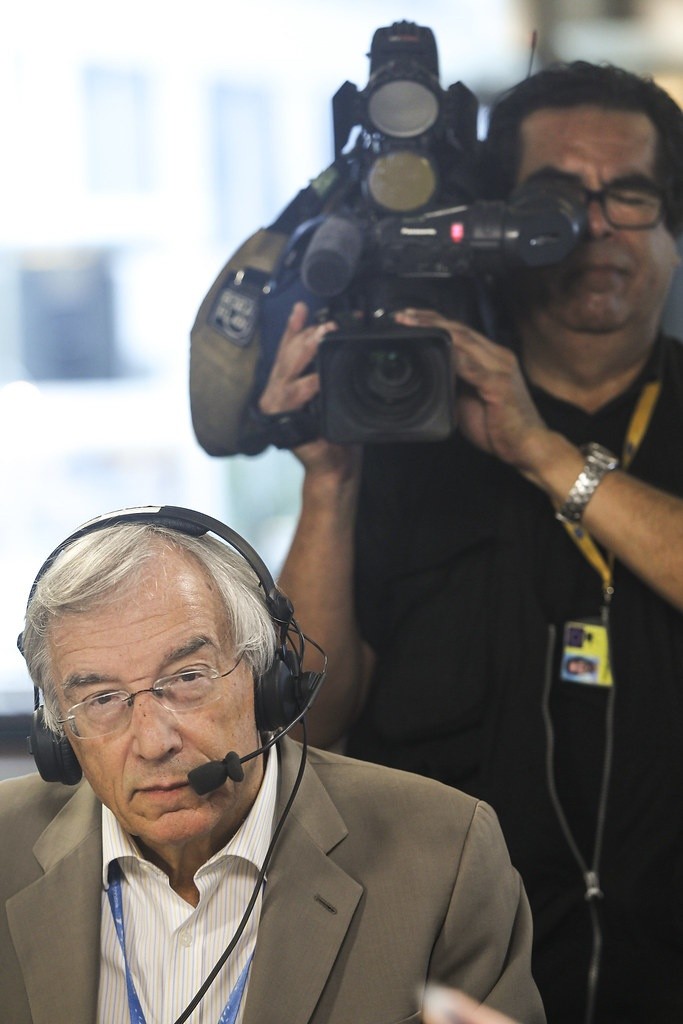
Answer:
[297,202,368,301]
[186,671,328,796]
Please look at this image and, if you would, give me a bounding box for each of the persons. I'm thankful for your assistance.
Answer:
[0,504,545,1024]
[259,61,682,1024]
[567,657,599,683]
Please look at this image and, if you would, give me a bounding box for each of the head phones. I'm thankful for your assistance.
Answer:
[14,503,318,784]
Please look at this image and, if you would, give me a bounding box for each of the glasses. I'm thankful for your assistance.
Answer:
[509,175,672,230]
[42,635,253,740]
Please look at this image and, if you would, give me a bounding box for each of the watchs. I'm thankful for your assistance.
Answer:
[554,443,618,523]
